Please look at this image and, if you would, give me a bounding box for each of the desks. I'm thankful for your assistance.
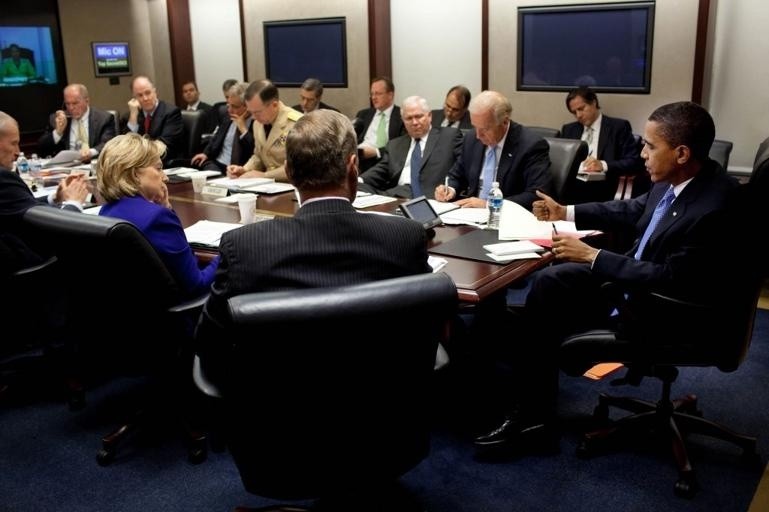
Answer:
[27,163,560,426]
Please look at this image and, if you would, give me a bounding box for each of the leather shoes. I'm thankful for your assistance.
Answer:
[476,419,544,446]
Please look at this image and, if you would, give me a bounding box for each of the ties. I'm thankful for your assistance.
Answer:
[478,146,496,198]
[447,120,455,127]
[411,138,422,198]
[584,127,592,144]
[143,112,152,135]
[612,186,676,316]
[377,109,387,147]
[78,119,90,156]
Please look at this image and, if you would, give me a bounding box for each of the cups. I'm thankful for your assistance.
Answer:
[237,193,258,224]
[191,174,207,193]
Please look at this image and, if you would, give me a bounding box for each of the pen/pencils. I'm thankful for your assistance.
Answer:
[552,223,558,235]
[445,177,448,193]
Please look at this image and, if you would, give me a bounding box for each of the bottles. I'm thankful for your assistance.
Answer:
[488,182,503,229]
[16,153,42,187]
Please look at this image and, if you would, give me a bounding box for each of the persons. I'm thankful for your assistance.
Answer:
[0,99,91,267]
[472,96,752,455]
[188,105,466,348]
[33,74,554,208]
[553,84,642,202]
[92,131,224,296]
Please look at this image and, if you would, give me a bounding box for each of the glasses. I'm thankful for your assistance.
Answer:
[61,102,81,117]
[227,102,245,108]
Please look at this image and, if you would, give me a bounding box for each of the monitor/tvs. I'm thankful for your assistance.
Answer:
[262,17,348,89]
[515,0,657,94]
[90,40,133,77]
[399,195,443,230]
[0,15,68,94]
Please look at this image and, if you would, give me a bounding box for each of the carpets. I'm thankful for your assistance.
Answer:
[1,299,767,509]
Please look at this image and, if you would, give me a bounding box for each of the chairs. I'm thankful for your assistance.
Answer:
[179,106,209,163]
